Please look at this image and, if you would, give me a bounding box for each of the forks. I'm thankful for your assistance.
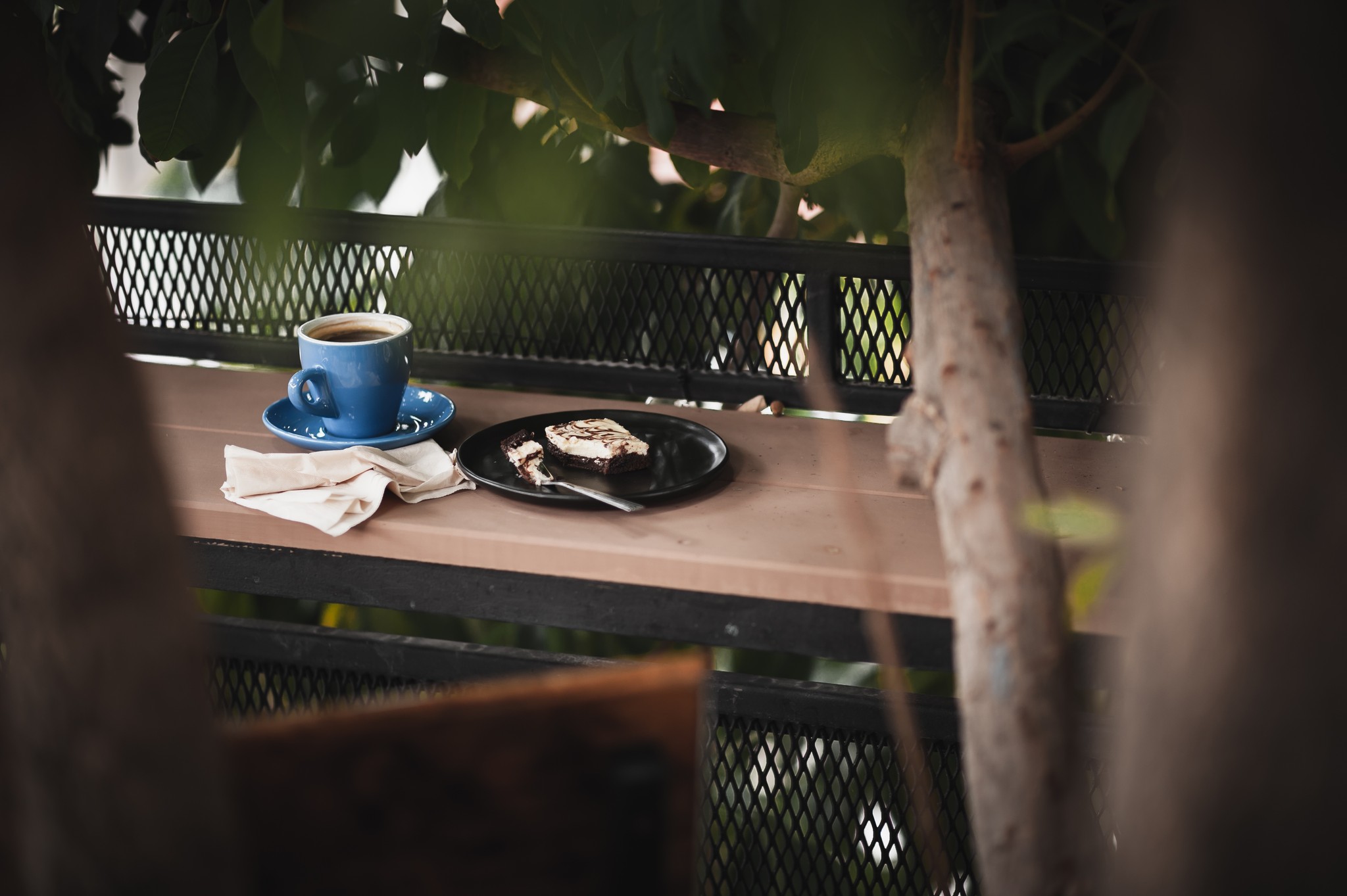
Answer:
[528,462,645,513]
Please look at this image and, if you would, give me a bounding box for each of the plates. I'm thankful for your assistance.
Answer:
[457,409,730,501]
[261,385,455,452]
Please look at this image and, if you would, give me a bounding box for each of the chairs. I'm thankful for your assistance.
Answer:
[224,648,708,896]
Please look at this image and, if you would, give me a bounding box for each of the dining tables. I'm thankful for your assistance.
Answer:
[127,345,1127,684]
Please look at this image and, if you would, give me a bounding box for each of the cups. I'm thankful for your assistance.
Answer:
[288,312,413,439]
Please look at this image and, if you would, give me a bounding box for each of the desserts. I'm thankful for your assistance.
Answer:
[546,418,652,474]
